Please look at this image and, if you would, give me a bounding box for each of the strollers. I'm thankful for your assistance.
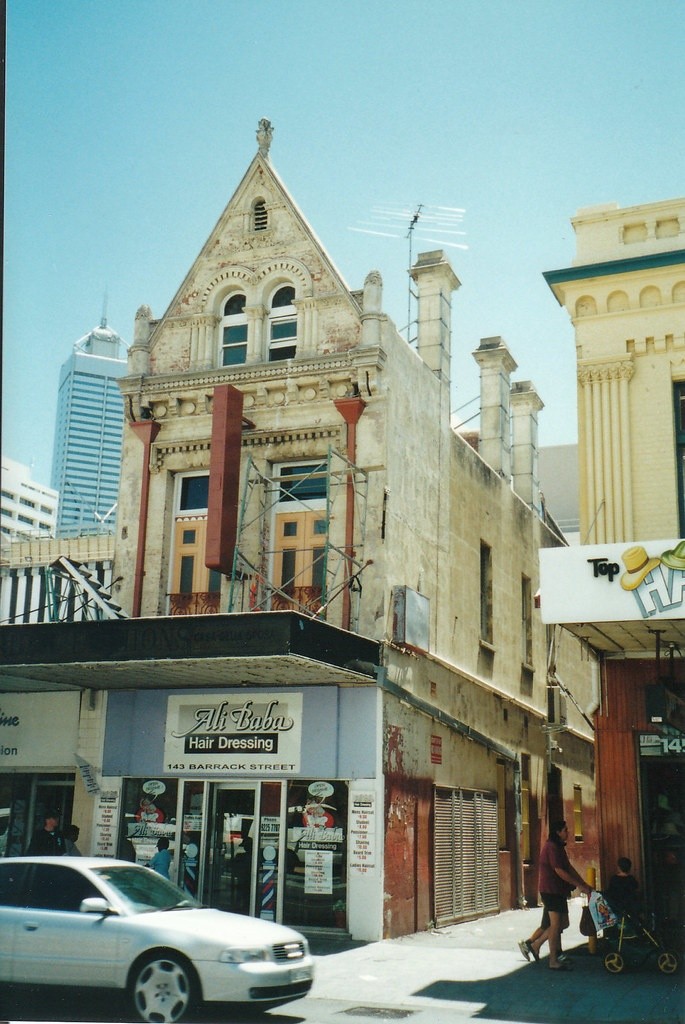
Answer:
[594,890,682,975]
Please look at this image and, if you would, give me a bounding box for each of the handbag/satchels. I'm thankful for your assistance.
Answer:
[588,892,618,931]
[580,905,596,936]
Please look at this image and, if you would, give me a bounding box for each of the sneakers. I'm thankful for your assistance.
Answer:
[519,941,530,959]
[556,950,573,963]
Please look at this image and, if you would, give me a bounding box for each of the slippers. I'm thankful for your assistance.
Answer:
[527,944,540,962]
[549,966,571,971]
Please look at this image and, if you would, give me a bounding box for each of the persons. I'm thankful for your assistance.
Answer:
[58,825,82,858]
[27,806,63,856]
[521,821,593,971]
[142,836,174,881]
[603,857,638,909]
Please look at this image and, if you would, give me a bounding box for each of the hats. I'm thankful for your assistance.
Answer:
[44,807,63,819]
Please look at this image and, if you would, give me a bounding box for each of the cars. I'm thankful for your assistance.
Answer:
[0,856,315,1023]
